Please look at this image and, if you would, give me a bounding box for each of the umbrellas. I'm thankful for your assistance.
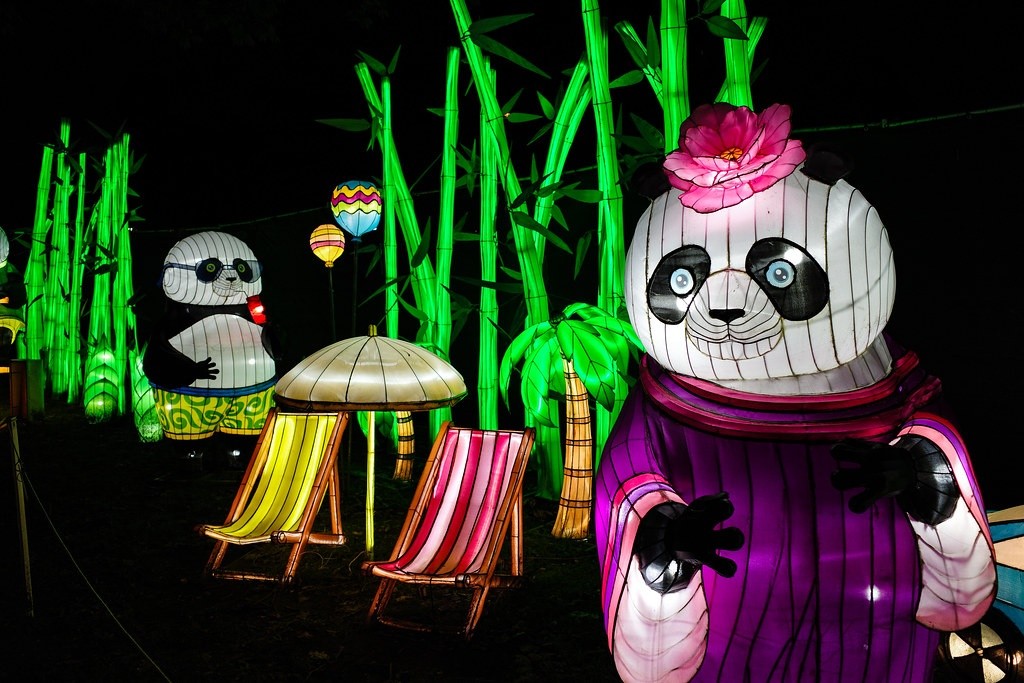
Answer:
[273,324,469,560]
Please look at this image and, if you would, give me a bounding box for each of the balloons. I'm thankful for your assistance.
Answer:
[330,180,382,242]
[309,223,345,267]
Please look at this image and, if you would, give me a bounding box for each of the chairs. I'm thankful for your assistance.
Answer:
[198,407,349,584]
[364,423,538,641]
